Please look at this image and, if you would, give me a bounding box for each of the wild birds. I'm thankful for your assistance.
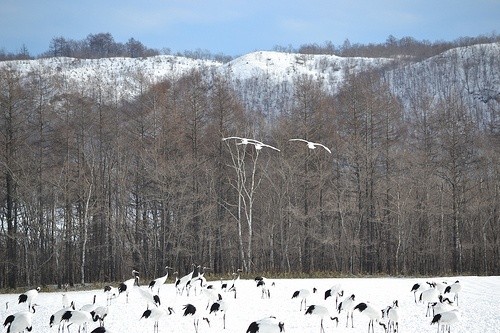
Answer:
[289,139,332,154]
[222,137,261,145]
[246,141,281,153]
[0,264,463,333]
